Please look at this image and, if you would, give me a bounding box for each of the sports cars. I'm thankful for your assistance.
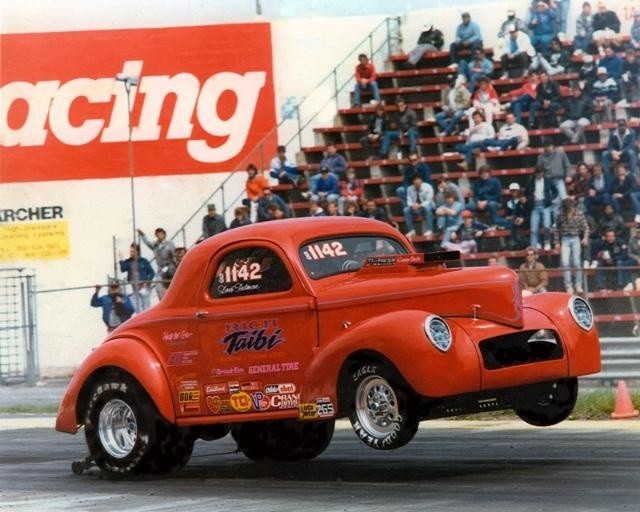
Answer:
[55,215,600,477]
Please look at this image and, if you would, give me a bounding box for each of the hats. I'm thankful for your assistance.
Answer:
[596,66,608,76]
[460,210,474,218]
[319,163,330,173]
[505,7,517,18]
[508,182,521,190]
[505,22,519,34]
[617,118,626,126]
[206,203,216,210]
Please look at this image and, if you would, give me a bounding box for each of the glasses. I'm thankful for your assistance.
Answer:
[526,253,535,257]
[263,192,272,196]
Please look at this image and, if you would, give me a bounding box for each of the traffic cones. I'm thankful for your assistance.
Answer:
[608,375,639,420]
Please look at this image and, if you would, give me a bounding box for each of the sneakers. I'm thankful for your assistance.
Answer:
[623,277,640,291]
[543,242,552,252]
[457,159,469,172]
[575,283,584,294]
[423,229,433,237]
[404,228,416,238]
[566,286,575,294]
[470,147,483,158]
[486,145,502,152]
[583,259,598,269]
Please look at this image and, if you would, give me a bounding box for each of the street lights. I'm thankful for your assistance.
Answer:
[115,72,140,245]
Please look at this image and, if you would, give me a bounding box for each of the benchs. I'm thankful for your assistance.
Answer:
[269,47,640,323]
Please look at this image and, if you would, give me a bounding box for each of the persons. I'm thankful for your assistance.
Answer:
[90,0,640,334]
